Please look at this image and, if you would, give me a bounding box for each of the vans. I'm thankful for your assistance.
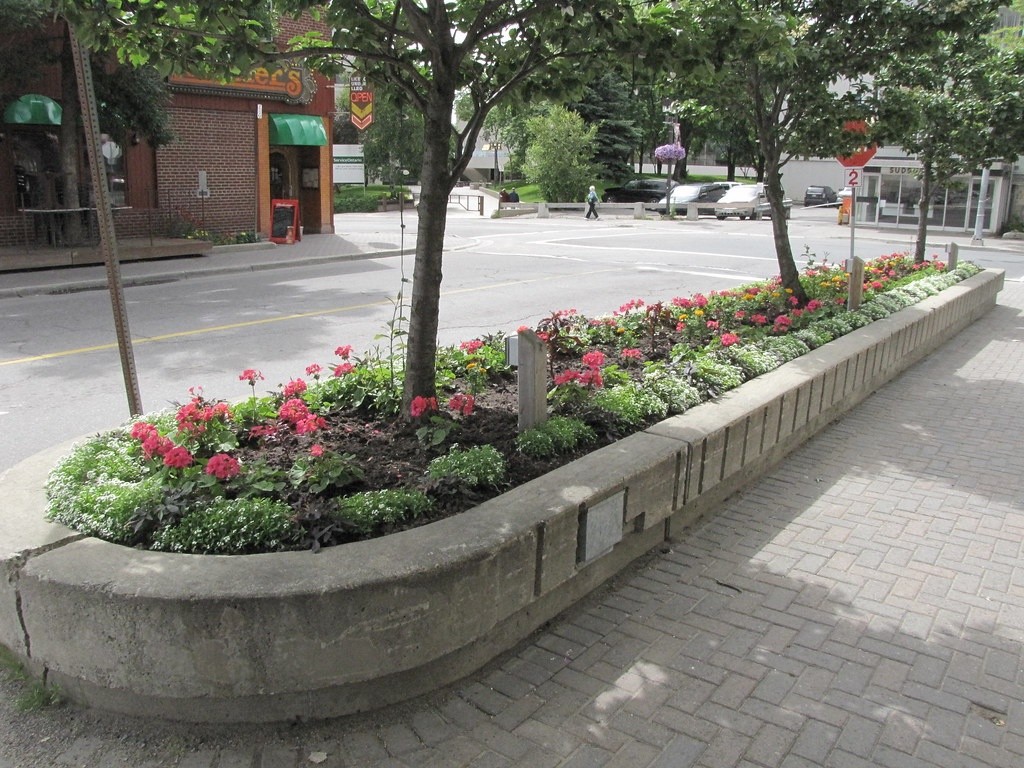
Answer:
[712,182,744,195]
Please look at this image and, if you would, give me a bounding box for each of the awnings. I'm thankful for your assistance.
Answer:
[269,112,329,146]
[0,92,66,126]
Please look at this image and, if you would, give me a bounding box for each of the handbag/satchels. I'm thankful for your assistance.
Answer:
[588,196,597,205]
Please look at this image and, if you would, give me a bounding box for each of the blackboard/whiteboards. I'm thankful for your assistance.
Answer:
[269,199,298,244]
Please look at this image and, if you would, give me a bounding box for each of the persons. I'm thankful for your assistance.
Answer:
[499,187,519,209]
[583,185,601,221]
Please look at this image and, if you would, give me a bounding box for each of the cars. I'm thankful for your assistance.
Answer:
[836,185,854,209]
[713,183,772,220]
[656,183,726,215]
[804,185,838,207]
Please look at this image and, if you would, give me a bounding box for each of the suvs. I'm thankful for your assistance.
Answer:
[600,177,680,204]
